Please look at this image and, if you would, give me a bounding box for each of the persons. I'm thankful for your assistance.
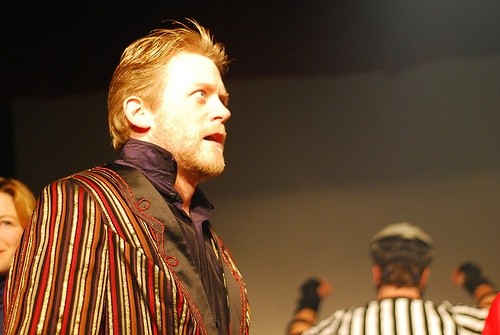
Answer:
[0,178,37,335]
[285,222,500,335]
[2,16,251,335]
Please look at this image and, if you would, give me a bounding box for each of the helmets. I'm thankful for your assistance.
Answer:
[368,222,435,263]
